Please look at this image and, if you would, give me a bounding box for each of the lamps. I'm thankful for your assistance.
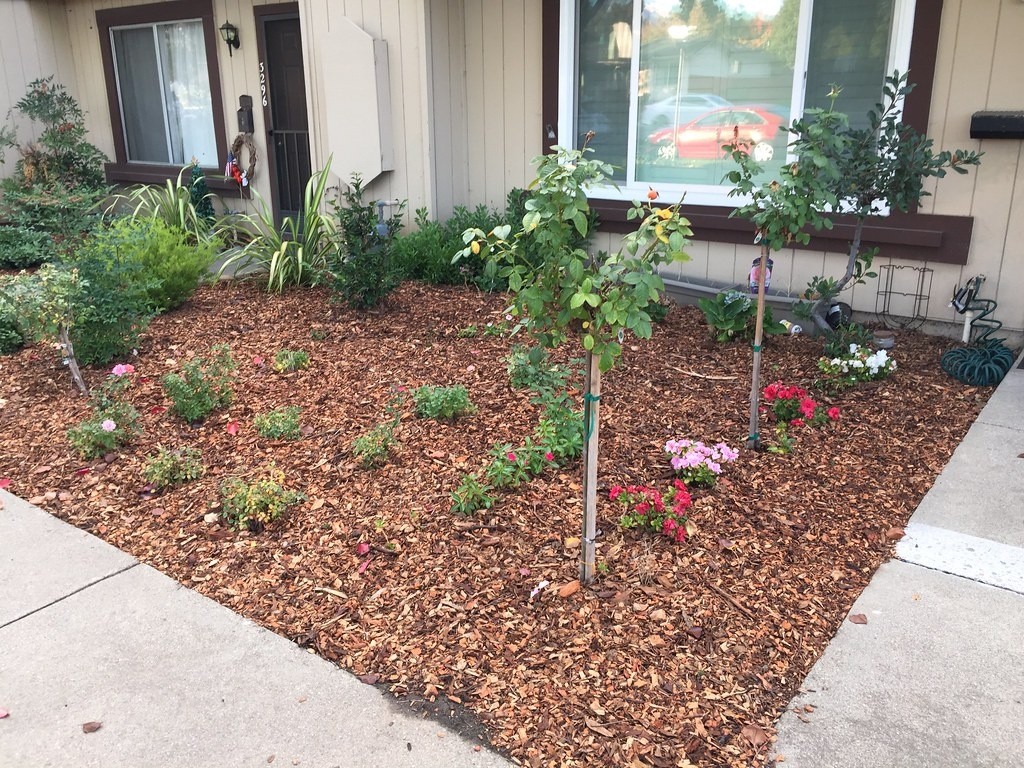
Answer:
[220,20,240,57]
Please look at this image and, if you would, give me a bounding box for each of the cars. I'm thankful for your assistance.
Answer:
[578,114,627,146]
[644,102,789,164]
[170,71,213,126]
[642,91,735,127]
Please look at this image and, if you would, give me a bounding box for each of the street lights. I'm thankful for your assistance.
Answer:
[667,23,689,167]
